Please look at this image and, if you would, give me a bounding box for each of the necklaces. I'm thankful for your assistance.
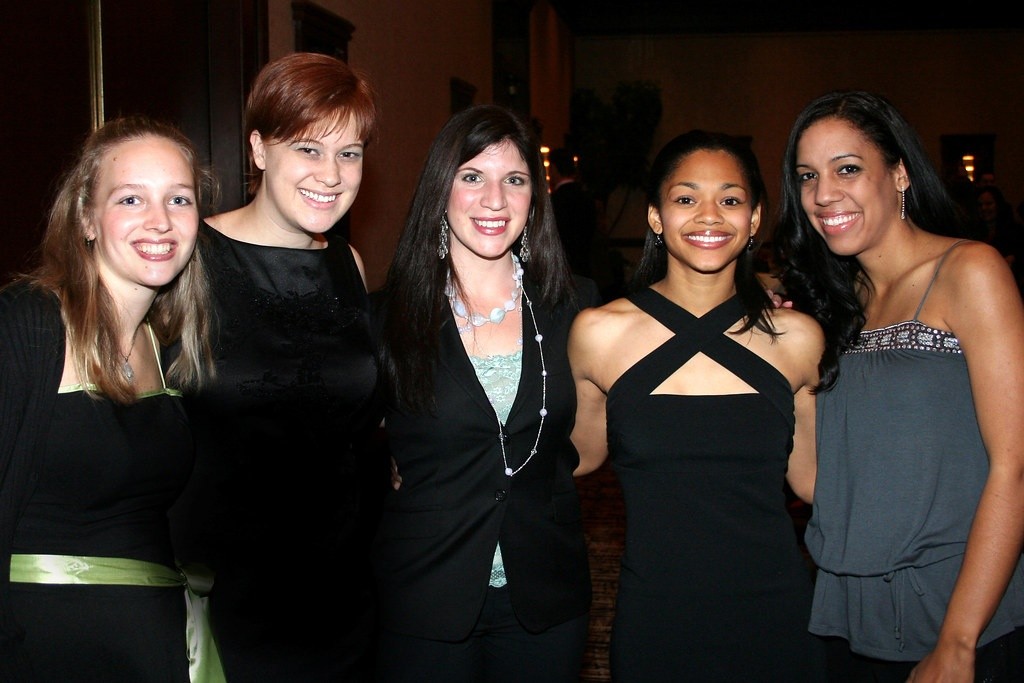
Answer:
[445,252,545,476]
[119,328,139,378]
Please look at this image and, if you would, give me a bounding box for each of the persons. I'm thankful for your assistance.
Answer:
[0,118,226,683]
[198,54,393,683]
[549,52,1024,683]
[377,103,591,683]
